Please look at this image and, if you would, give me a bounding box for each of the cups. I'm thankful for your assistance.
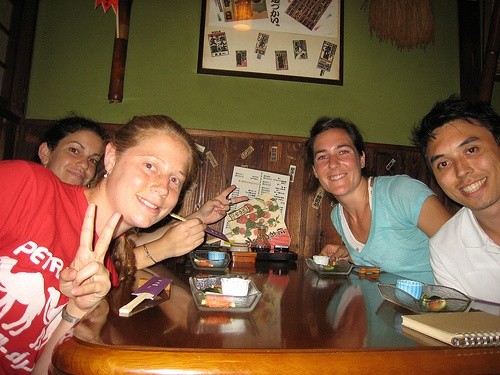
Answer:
[207,251,226,266]
[274,245,289,253]
[219,278,252,296]
[395,279,424,302]
[312,255,329,266]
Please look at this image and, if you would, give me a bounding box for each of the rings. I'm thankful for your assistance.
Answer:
[228,199,232,204]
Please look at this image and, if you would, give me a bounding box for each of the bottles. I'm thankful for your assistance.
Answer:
[251,225,270,253]
[230,243,249,252]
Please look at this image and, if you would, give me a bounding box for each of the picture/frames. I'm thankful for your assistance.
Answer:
[197,0,345,87]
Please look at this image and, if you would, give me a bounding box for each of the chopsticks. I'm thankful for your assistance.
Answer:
[118,293,149,313]
[169,212,189,222]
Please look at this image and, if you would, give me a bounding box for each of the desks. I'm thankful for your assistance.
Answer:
[51,247,500,375]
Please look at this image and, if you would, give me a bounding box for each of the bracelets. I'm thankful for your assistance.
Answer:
[142,244,158,265]
[61,306,80,325]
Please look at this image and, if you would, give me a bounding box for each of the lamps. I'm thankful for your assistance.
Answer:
[232,0,254,31]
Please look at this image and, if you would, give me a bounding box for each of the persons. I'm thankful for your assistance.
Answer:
[407,95,500,305]
[308,116,454,286]
[0,115,248,375]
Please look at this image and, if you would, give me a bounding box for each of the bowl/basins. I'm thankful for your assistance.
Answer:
[190,249,231,271]
[376,282,471,314]
[189,273,262,313]
[305,258,354,275]
